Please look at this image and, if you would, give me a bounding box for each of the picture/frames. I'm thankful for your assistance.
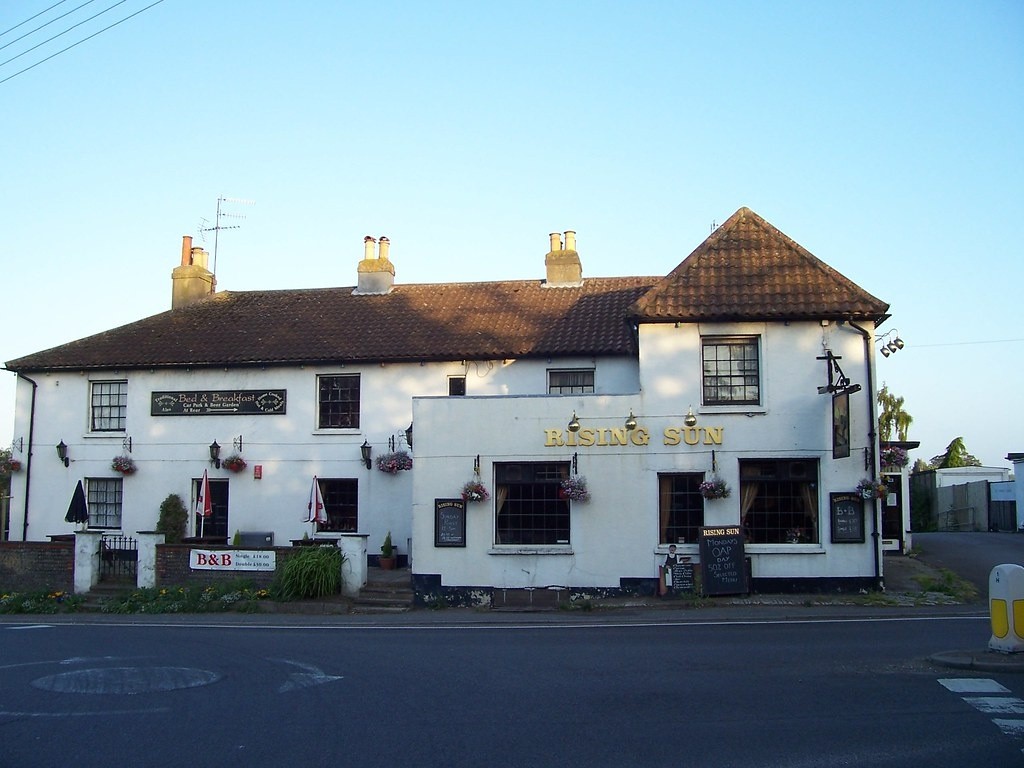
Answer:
[829,491,867,543]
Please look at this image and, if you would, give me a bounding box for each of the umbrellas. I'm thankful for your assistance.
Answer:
[65,480,89,532]
[300,475,327,539]
[196,469,212,537]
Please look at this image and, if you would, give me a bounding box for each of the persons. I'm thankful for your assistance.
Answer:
[661,545,688,591]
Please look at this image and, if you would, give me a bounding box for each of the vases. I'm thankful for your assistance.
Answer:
[122,470,130,474]
[230,464,240,472]
[705,490,716,498]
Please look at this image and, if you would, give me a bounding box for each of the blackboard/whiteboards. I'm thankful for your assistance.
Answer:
[151,390,286,416]
[830,492,865,543]
[434,499,466,547]
[700,526,748,595]
[673,564,693,593]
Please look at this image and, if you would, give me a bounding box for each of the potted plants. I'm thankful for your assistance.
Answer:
[378,531,395,570]
[299,531,314,546]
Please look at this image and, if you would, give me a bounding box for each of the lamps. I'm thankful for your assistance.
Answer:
[404,422,413,451]
[568,408,580,432]
[56,438,70,467]
[625,407,640,429]
[360,436,373,470]
[208,437,221,469]
[683,403,698,426]
[875,328,905,358]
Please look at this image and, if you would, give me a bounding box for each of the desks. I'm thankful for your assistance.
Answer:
[182,536,232,544]
[288,536,341,547]
[45,533,75,542]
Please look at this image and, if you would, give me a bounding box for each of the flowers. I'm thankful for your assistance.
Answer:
[459,480,490,504]
[854,472,892,501]
[222,455,248,471]
[698,476,731,501]
[111,455,137,475]
[560,469,593,504]
[0,451,21,475]
[881,445,911,468]
[784,526,808,543]
[377,451,412,474]
